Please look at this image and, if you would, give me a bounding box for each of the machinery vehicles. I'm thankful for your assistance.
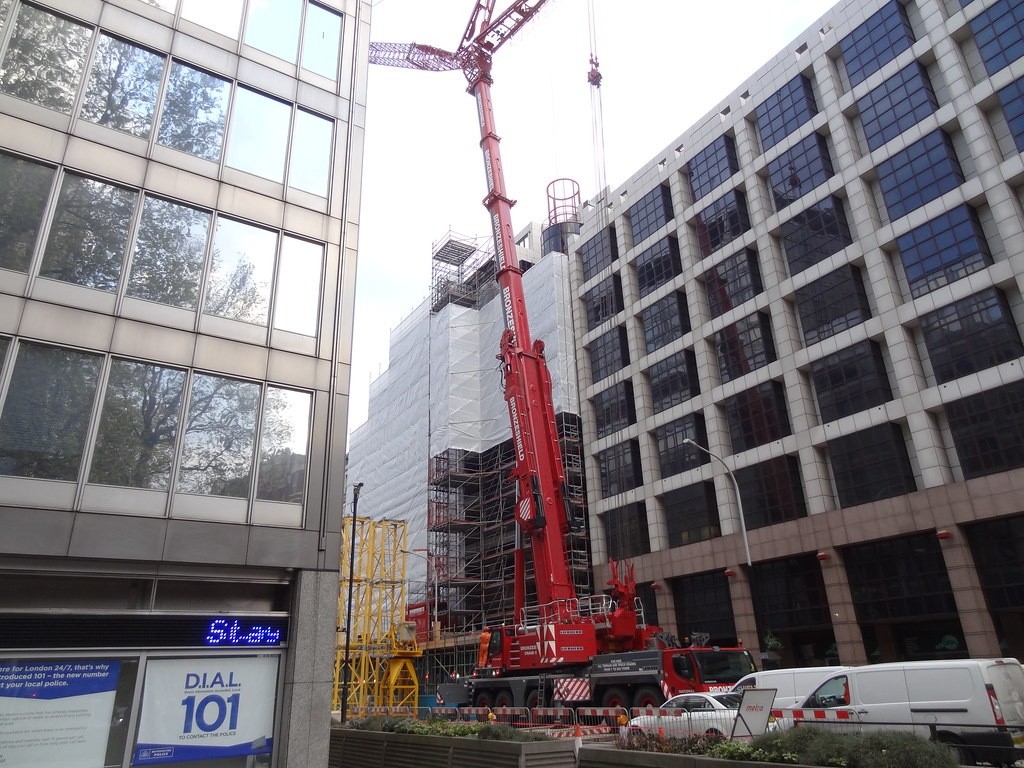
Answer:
[368,0,757,734]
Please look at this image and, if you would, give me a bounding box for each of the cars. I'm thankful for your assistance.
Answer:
[619,693,777,749]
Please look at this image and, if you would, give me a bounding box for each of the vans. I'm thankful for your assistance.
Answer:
[727,666,854,732]
[773,658,1024,766]
